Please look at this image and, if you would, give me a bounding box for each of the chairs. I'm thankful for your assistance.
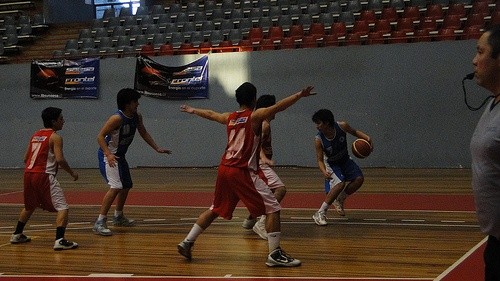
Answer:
[0,1,48,64]
[50,0,500,61]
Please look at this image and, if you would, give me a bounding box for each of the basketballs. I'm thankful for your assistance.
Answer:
[351,138,373,159]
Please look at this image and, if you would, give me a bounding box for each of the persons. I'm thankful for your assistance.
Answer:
[10,107,78,250]
[312,109,372,226]
[178,82,317,268]
[242,94,286,239]
[92,88,172,235]
[470,23,500,281]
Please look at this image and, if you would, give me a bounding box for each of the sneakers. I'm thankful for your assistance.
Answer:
[332,199,345,216]
[92,217,113,236]
[311,210,328,226]
[53,237,79,251]
[9,233,32,244]
[177,240,192,259]
[112,211,135,227]
[241,217,261,229]
[264,245,302,267]
[252,218,268,241]
